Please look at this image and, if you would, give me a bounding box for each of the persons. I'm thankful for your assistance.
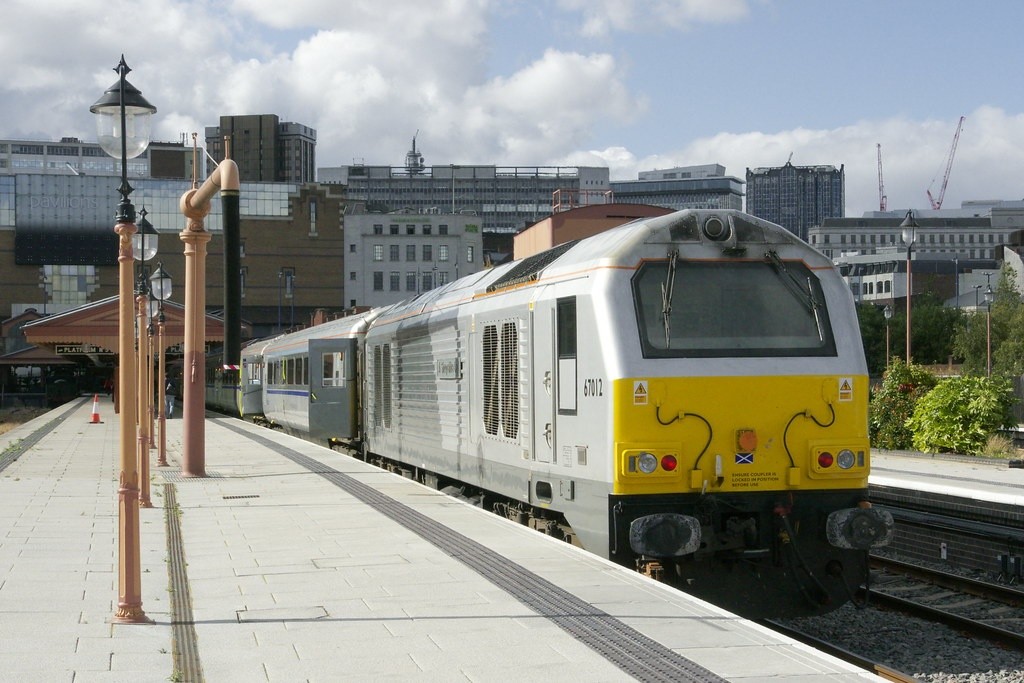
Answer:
[164,373,176,419]
[104,376,113,396]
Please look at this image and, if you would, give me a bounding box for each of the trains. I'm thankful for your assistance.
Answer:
[165,207,895,615]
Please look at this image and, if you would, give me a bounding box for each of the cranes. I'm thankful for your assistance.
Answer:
[925,115,969,211]
[876,142,887,212]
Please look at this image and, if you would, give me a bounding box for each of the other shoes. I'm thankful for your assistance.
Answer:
[167,413,172,419]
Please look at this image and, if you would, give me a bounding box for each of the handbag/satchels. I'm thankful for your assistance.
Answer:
[166,380,175,395]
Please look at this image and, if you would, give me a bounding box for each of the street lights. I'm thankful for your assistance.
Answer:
[132,204,162,509]
[983,284,994,381]
[883,303,894,371]
[87,51,160,625]
[133,319,140,425]
[897,209,920,370]
[980,271,995,287]
[971,284,982,312]
[148,261,173,469]
[143,287,159,449]
[951,258,960,308]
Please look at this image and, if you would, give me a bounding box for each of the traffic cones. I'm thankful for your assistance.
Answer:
[88,394,105,424]
